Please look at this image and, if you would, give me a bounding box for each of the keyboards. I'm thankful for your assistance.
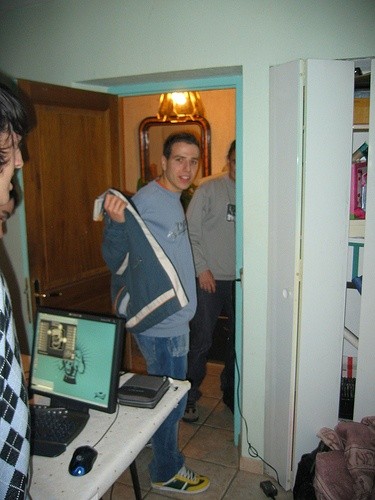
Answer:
[28,404,90,458]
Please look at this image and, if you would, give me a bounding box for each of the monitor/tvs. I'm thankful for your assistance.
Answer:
[27,305,126,415]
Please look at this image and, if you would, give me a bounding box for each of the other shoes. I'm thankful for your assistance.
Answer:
[181,399,199,422]
[221,390,235,414]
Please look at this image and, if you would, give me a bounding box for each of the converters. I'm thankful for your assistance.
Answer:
[260,480,278,497]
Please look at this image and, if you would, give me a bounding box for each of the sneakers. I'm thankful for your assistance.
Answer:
[150,464,210,494]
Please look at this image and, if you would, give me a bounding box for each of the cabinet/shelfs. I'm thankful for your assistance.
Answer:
[262,56,375,491]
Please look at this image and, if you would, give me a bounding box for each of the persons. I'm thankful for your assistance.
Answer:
[100,131,211,495]
[181,139,237,422]
[0,70,37,500]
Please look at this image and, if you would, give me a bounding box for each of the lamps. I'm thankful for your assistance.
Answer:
[156,91,205,123]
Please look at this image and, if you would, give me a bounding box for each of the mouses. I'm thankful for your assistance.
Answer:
[69,446,98,476]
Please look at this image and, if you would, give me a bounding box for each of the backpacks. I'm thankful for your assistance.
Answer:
[293,440,331,500]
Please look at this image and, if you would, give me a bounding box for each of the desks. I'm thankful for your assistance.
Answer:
[20,354,191,500]
